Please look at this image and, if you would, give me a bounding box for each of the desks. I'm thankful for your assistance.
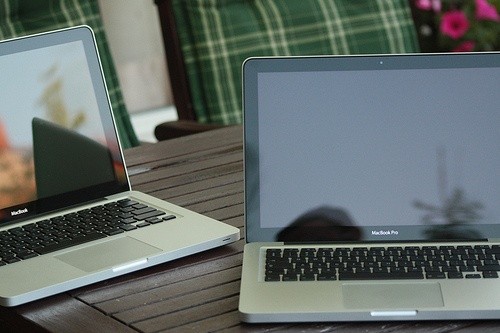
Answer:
[0,123,499,333]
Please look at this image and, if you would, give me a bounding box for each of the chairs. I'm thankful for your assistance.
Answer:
[154,0,422,141]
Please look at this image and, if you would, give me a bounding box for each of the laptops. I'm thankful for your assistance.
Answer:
[237,50,499,321]
[0,23,239,309]
